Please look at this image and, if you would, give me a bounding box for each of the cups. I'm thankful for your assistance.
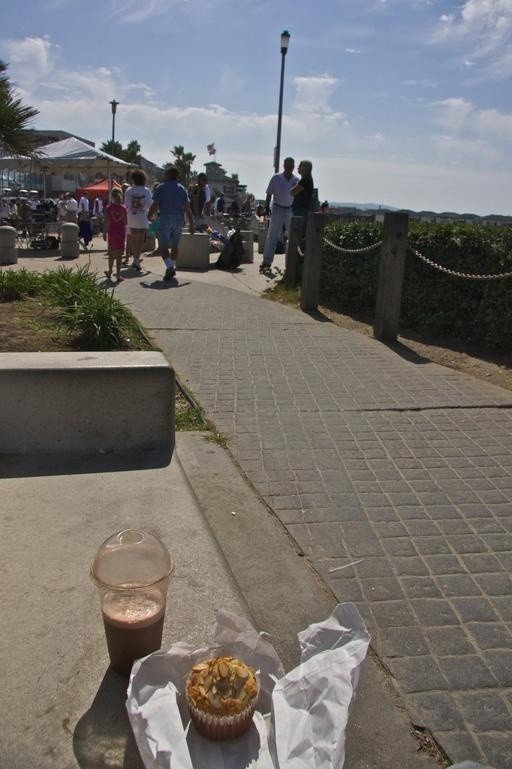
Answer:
[90,529,173,673]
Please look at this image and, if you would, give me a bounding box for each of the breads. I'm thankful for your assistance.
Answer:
[185,655,261,741]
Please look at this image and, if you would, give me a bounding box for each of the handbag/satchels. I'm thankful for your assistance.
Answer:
[150,220,160,235]
[310,188,320,212]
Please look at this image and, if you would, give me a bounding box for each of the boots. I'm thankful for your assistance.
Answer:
[163,267,176,281]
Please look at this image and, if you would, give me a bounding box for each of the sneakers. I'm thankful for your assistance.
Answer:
[131,257,143,271]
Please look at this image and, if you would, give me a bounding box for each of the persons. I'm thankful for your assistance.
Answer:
[0,166,226,282]
[281,160,315,287]
[321,200,329,213]
[259,157,299,274]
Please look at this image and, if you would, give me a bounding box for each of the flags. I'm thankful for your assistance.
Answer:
[207,144,216,156]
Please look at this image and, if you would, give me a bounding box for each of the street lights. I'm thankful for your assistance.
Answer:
[109,99,119,154]
[273,30,290,173]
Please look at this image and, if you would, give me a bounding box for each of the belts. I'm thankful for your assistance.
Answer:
[273,203,294,208]
